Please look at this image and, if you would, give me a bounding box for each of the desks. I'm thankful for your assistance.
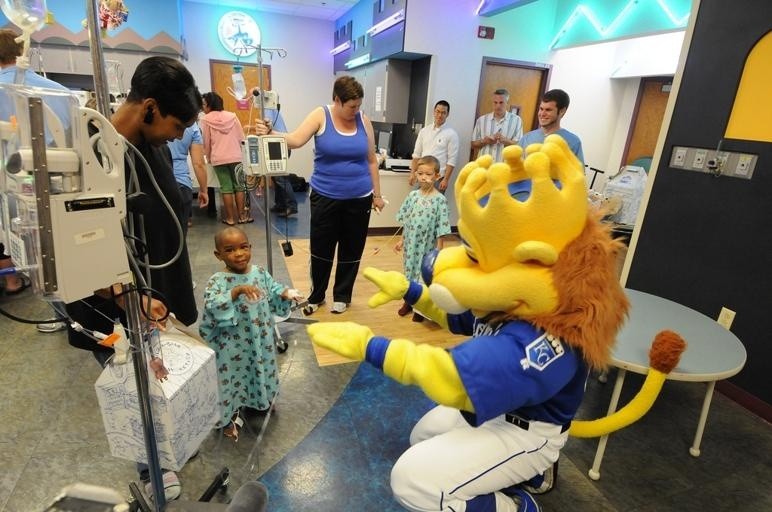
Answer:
[585,284,747,481]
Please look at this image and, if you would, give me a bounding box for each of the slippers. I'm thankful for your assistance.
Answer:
[7,277,31,294]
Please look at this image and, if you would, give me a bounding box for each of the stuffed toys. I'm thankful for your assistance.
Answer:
[305,134,688,512]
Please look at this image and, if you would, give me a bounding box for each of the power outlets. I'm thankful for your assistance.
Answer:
[670,147,758,184]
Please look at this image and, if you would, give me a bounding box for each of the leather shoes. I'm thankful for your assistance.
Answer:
[279,207,298,216]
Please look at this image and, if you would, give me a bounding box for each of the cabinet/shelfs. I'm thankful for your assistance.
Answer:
[373,0,431,64]
[330,0,373,76]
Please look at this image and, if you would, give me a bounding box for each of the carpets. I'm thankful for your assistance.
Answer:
[278,235,472,368]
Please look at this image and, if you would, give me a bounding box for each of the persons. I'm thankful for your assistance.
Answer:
[167,87,298,226]
[394,100,459,322]
[471,89,523,161]
[197,225,305,442]
[65,56,203,505]
[518,89,584,163]
[254,76,385,313]
[1,29,127,333]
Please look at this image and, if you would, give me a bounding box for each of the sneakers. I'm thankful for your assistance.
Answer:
[222,217,254,226]
[331,302,346,312]
[308,299,325,311]
